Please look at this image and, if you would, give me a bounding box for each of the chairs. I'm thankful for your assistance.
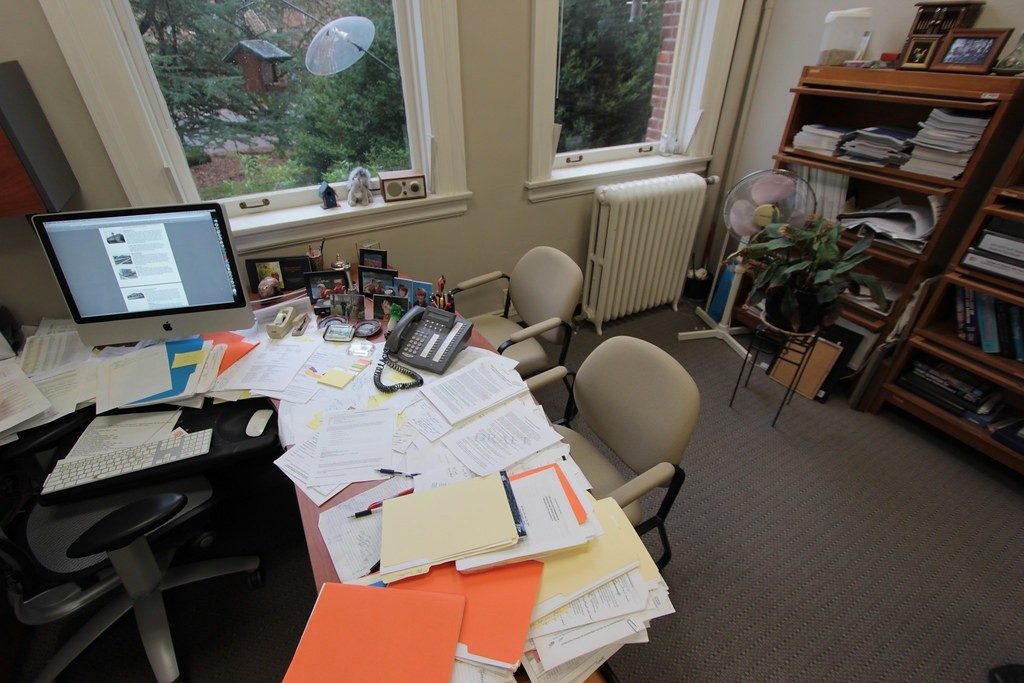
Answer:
[445,243,584,420]
[523,334,701,574]
[0,397,263,683]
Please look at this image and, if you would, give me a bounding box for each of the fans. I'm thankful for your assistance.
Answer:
[678,169,819,362]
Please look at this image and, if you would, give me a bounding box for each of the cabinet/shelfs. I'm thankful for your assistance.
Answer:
[751,64,1024,475]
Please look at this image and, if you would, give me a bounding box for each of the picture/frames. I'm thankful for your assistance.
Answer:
[929,27,1015,74]
[302,249,398,318]
[896,34,942,70]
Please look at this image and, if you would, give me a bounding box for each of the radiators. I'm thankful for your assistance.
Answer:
[582,174,717,335]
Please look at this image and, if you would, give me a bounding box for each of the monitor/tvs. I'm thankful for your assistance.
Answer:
[31,201,255,346]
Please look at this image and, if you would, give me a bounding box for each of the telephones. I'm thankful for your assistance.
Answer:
[385,305,474,375]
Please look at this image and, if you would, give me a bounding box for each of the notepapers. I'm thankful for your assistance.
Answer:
[318,369,355,388]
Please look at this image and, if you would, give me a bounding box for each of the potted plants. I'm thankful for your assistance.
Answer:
[725,206,887,333]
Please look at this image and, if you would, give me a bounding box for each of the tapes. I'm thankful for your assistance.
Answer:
[275,313,286,323]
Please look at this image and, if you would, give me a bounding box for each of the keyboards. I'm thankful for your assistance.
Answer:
[40,428,214,495]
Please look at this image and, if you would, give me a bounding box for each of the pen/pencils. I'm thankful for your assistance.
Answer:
[308,237,325,256]
[367,488,414,510]
[430,292,453,309]
[375,468,421,478]
[347,506,382,519]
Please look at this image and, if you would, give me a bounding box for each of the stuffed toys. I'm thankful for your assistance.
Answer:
[345,166,373,207]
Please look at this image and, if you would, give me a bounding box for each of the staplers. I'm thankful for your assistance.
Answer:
[292,313,311,336]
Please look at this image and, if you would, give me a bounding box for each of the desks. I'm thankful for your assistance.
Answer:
[0,262,568,683]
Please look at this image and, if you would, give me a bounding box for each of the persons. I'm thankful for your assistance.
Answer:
[271,271,284,288]
[914,42,1021,67]
[318,278,428,321]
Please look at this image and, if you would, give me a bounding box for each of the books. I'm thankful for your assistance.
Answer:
[782,106,1024,448]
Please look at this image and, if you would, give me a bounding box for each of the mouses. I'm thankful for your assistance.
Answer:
[246,410,273,437]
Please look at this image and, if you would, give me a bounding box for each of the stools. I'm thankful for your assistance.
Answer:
[728,313,818,427]
